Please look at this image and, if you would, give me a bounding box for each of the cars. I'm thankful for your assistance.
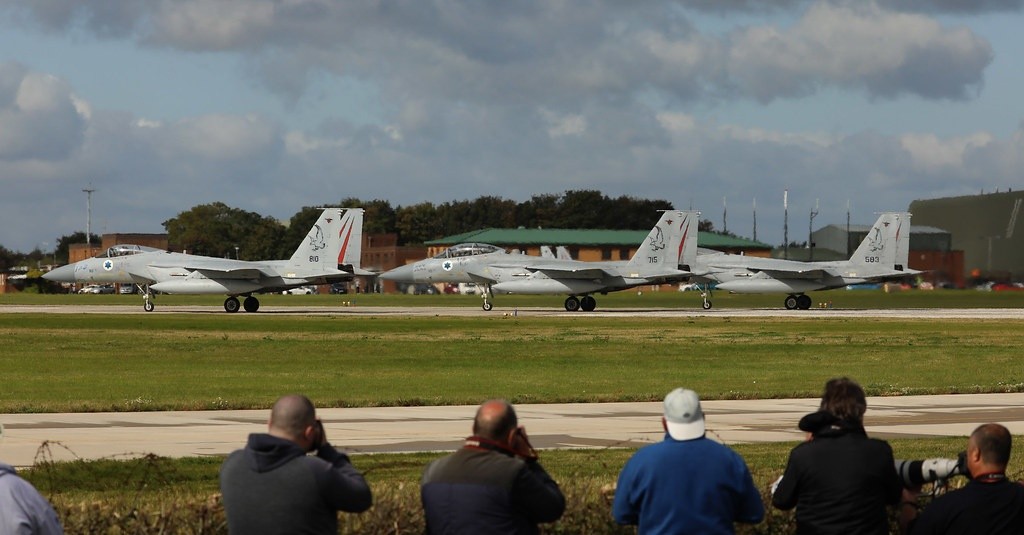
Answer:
[282,286,311,294]
[78,285,98,294]
[330,284,347,294]
[90,284,116,294]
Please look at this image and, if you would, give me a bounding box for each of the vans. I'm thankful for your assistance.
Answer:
[119,283,133,294]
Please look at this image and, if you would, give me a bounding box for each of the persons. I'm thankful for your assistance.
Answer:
[0,463,63,535]
[612,387,765,535]
[420,401,565,535]
[771,376,1024,535]
[220,395,373,535]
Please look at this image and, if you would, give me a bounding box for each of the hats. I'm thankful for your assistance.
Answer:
[663,388,705,441]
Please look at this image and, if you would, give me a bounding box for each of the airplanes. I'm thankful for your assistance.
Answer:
[40,208,375,312]
[680,212,924,310]
[377,209,725,311]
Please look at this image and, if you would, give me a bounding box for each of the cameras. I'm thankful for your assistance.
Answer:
[895,451,973,490]
